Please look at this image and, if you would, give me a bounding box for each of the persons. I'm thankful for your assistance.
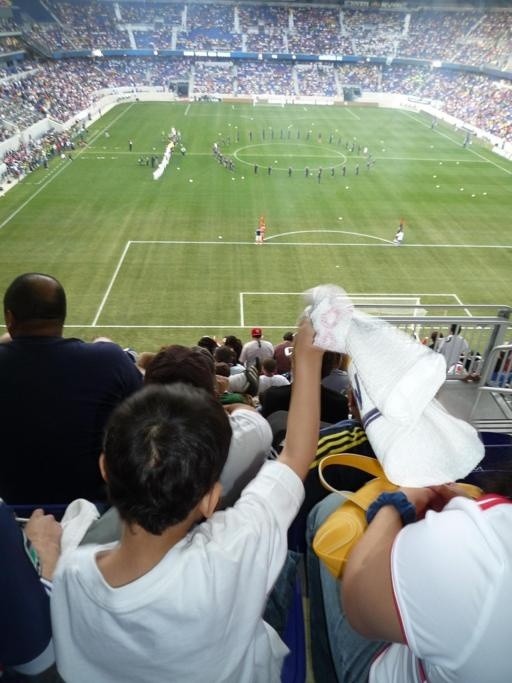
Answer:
[393,218,405,244]
[0,2,512,183]
[255,215,266,246]
[1,273,511,683]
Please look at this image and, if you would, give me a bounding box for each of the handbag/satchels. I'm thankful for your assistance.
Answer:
[313,475,486,579]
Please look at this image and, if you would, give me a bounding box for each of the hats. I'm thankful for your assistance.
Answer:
[251,329,262,337]
